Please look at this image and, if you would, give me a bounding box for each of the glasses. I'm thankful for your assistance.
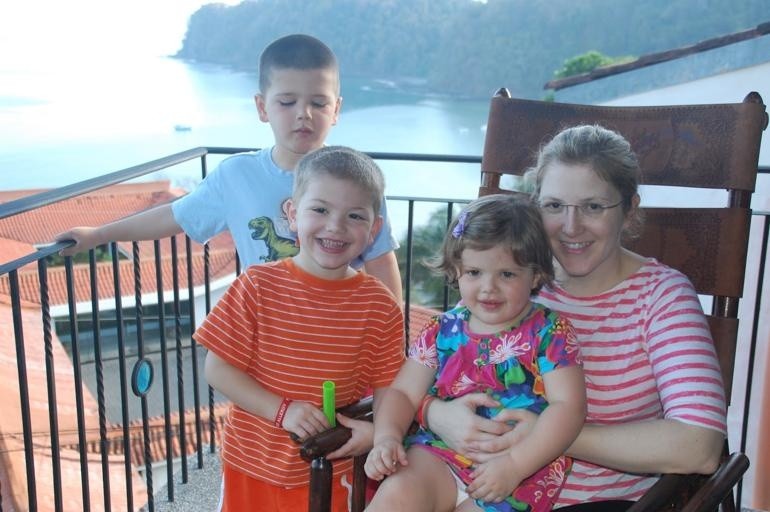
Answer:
[529,191,628,222]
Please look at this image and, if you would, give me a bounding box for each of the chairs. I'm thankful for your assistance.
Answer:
[291,86,769,512]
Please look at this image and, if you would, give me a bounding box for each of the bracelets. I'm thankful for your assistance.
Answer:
[273,396,294,429]
[421,395,440,431]
[416,394,435,427]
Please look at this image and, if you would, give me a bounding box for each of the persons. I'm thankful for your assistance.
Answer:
[410,121,730,512]
[355,190,589,512]
[187,144,410,511]
[53,32,407,319]
